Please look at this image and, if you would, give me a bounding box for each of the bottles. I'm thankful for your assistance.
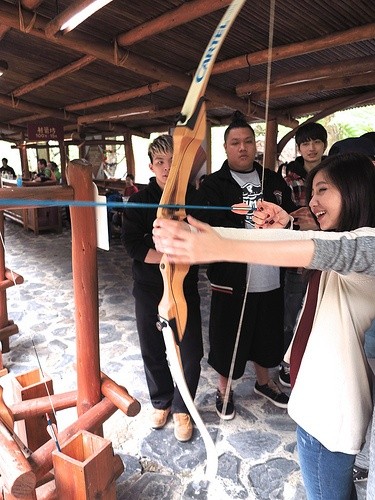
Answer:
[17,175,22,187]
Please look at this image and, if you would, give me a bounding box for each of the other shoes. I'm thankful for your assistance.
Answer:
[254,379,289,408]
[215,387,235,420]
[172,413,193,441]
[151,406,171,428]
[353,465,368,482]
[279,364,291,387]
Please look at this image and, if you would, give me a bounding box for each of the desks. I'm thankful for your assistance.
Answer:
[0,176,62,235]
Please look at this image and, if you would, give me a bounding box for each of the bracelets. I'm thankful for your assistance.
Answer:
[285,220,291,230]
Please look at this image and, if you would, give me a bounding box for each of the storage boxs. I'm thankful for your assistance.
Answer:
[51,430,118,500]
[12,368,58,452]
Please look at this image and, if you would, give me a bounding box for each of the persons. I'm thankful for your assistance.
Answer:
[151,152,375,500]
[120,135,213,443]
[28,159,61,182]
[124,174,138,197]
[278,121,328,388]
[328,137,375,167]
[0,158,17,179]
[198,110,289,421]
